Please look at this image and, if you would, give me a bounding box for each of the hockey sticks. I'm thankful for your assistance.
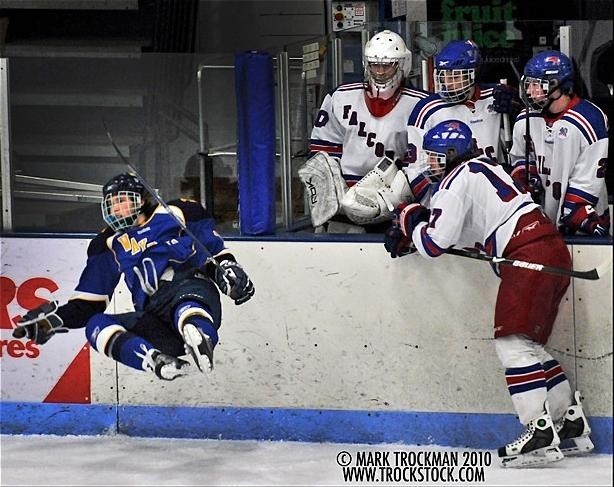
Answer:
[403,241,613,280]
[509,59,529,191]
[102,116,236,287]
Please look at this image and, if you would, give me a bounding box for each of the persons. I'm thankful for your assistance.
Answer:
[385,121,596,458]
[12,172,255,381]
[304,30,614,243]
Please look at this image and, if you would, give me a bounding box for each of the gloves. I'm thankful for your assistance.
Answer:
[12,301,69,345]
[215,259,255,305]
[565,204,610,237]
[384,202,429,258]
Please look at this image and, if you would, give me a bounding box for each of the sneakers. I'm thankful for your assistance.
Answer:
[147,352,190,381]
[498,389,592,457]
[183,320,213,373]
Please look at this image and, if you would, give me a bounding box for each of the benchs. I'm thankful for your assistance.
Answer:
[0,0,155,234]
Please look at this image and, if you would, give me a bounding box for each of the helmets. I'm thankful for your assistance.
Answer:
[519,50,575,110]
[423,120,472,184]
[362,30,412,100]
[434,40,480,103]
[101,174,144,230]
